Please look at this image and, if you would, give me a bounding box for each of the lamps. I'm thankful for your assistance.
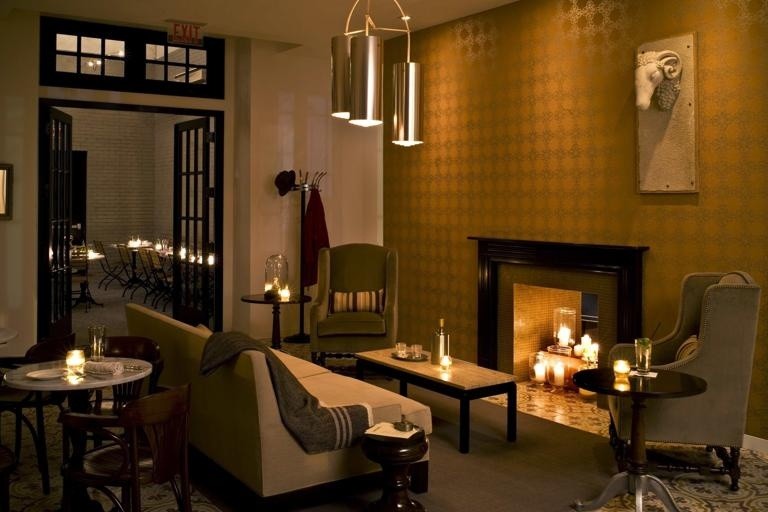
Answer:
[333,0,423,150]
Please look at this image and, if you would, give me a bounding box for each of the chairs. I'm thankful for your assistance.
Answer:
[70,245,89,312]
[56,336,164,477]
[94,239,202,312]
[0,331,76,470]
[59,385,190,512]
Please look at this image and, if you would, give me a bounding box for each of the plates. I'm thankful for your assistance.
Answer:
[392,352,428,362]
[26,368,70,380]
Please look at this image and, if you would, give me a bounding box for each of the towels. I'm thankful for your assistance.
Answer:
[84,361,124,378]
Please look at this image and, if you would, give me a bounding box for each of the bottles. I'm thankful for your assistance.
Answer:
[432,319,450,365]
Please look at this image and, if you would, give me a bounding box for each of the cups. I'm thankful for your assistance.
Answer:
[441,355,451,369]
[635,338,653,373]
[88,325,105,362]
[410,344,423,360]
[280,289,290,302]
[65,351,86,367]
[131,234,140,247]
[395,343,407,358]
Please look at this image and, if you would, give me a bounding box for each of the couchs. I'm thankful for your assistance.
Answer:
[308,242,399,368]
[605,268,761,489]
[125,301,434,512]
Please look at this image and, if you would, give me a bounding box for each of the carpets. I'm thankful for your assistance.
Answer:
[559,439,768,512]
[0,393,224,512]
[337,366,662,511]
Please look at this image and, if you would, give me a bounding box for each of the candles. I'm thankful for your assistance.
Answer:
[574,335,598,356]
[555,363,565,386]
[66,348,84,372]
[278,284,290,302]
[558,327,571,347]
[535,360,546,384]
[441,356,451,371]
[438,316,445,335]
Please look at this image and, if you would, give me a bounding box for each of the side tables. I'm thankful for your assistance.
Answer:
[573,367,705,507]
[242,292,311,349]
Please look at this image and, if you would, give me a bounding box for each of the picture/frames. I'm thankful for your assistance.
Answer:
[0,162,14,221]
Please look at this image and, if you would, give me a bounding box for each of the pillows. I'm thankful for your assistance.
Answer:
[327,288,388,314]
